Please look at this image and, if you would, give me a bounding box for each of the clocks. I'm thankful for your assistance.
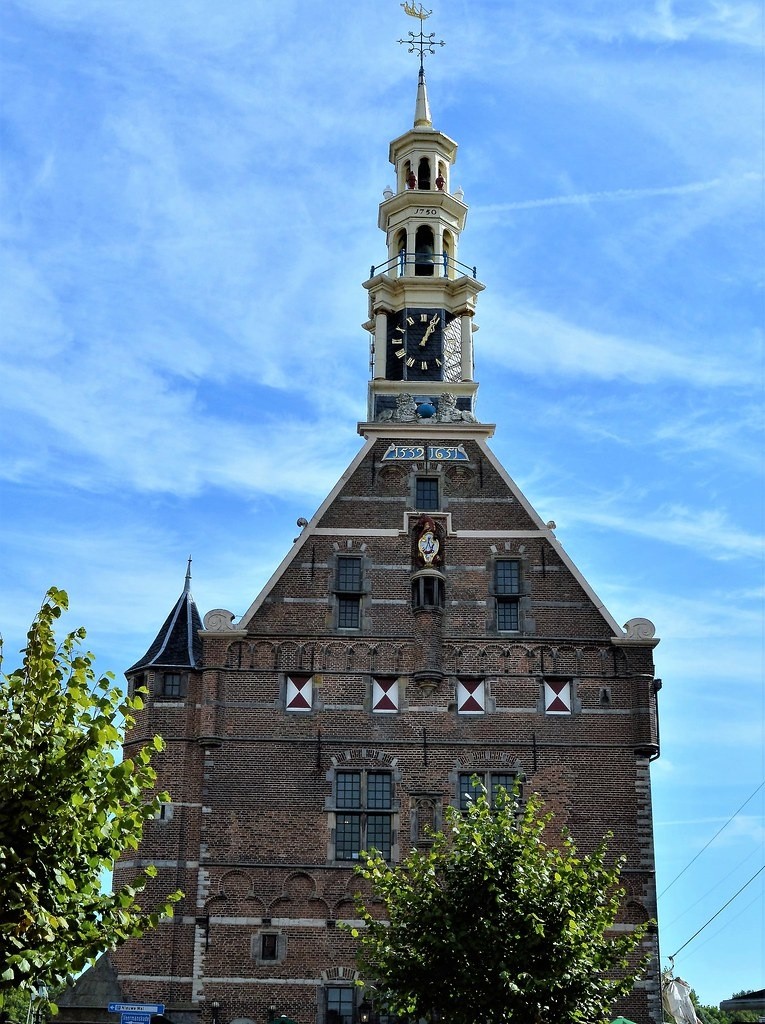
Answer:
[386,308,463,381]
